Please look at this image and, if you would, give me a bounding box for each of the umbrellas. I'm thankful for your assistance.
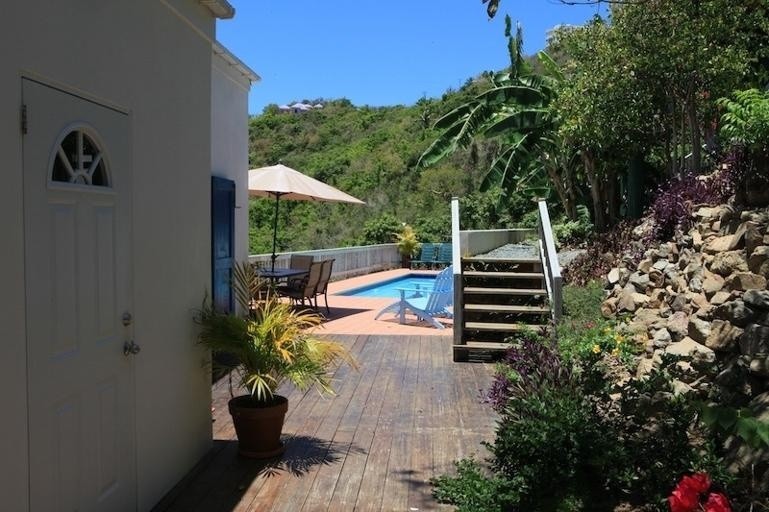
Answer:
[248,159,366,272]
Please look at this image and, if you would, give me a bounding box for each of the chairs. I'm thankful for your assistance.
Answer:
[374,265,454,328]
[255,260,281,300]
[434,242,452,266]
[274,261,322,314]
[410,242,434,271]
[274,254,314,309]
[292,259,336,313]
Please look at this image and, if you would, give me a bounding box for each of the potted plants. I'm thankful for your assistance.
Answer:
[189,256,359,453]
[394,222,421,268]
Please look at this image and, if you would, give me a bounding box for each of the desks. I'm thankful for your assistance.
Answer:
[251,267,310,298]
